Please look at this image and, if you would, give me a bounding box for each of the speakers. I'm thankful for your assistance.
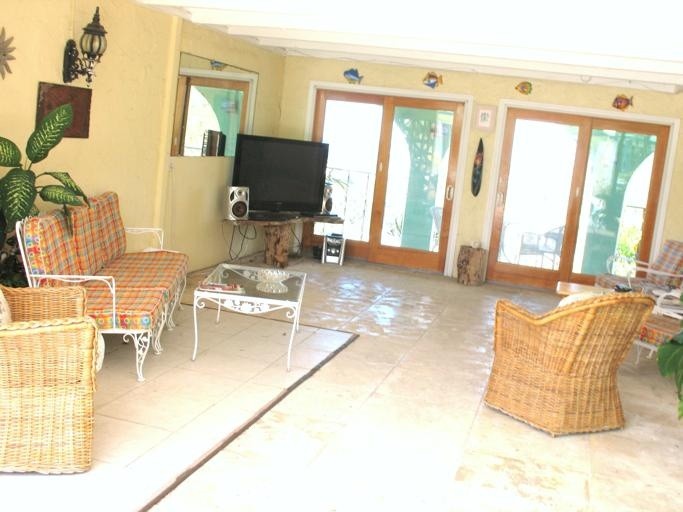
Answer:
[223,186,249,220]
[301,181,334,215]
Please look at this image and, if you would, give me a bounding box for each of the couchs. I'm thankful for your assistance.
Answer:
[14,187,188,381]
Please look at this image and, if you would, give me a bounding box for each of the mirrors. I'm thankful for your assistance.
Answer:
[170,68,260,158]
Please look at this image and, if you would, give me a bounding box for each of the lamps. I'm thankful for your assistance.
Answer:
[63,6,108,88]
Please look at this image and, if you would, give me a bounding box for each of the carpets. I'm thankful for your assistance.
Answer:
[1,300,360,512]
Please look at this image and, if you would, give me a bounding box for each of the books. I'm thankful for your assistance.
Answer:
[198,280,246,294]
[652,293,683,322]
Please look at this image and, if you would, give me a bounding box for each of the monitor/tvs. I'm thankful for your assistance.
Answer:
[231,133,329,221]
[206,130,227,156]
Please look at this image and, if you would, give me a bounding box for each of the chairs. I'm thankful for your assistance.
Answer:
[595,238,683,366]
[0,286,96,475]
[482,290,657,437]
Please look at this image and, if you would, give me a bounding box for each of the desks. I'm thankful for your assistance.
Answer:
[220,211,345,269]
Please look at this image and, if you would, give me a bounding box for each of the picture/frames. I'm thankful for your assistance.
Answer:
[475,104,496,132]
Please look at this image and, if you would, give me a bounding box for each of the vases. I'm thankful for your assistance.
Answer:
[325,197,333,215]
